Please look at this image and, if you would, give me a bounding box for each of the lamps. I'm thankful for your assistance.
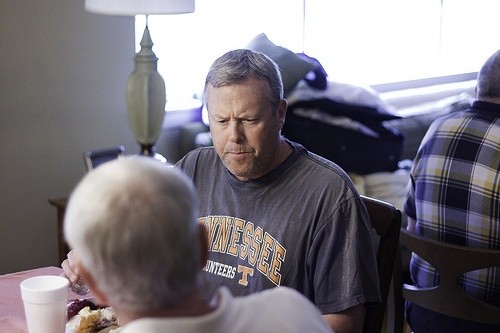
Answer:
[85,0,196,166]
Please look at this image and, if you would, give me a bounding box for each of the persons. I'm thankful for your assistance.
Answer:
[404,48,500,333]
[64,156,334,333]
[144,49,383,333]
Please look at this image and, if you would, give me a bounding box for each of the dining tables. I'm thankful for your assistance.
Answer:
[0,266,98,333]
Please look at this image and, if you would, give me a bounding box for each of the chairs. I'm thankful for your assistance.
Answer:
[385,229,500,333]
[355,193,402,333]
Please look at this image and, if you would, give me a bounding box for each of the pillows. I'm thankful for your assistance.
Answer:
[282,110,405,170]
[282,78,405,123]
[239,33,311,101]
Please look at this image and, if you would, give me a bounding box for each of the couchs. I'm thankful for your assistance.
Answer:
[181,80,481,204]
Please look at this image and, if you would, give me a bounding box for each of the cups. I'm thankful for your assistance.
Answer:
[20,275,70,333]
[67,250,90,296]
